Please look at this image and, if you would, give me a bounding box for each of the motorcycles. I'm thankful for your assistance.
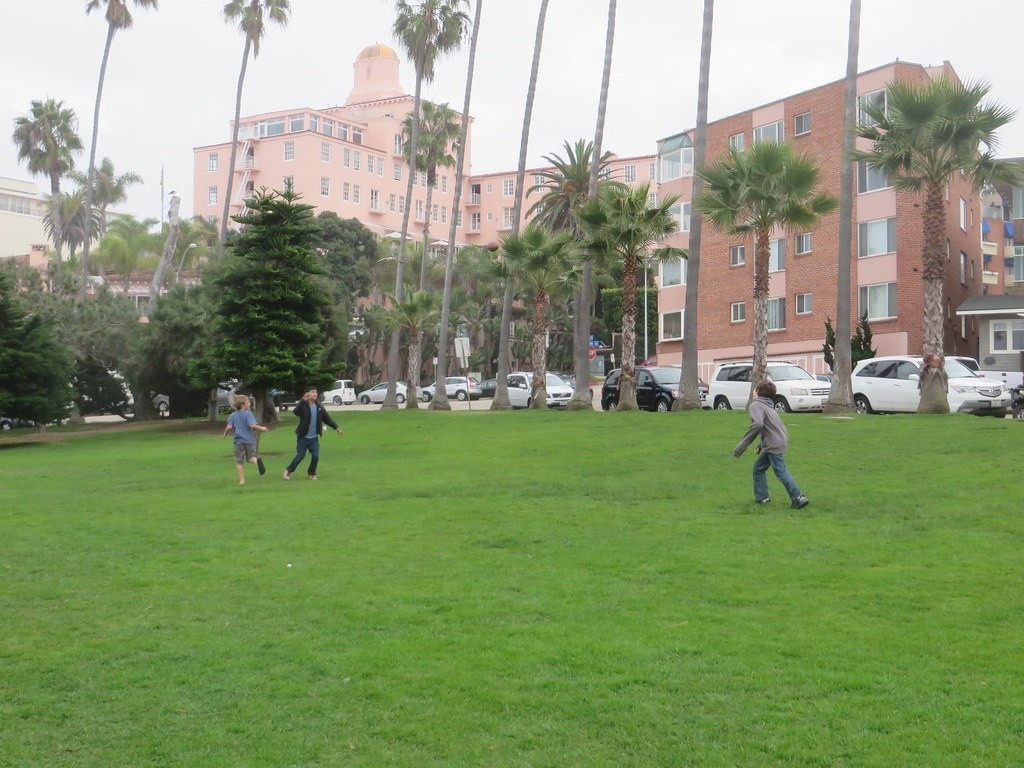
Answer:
[1010,385,1024,420]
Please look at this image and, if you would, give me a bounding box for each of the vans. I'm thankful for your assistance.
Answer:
[320,379,356,406]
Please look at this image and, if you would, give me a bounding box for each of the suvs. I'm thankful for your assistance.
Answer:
[705,360,833,413]
[851,354,1013,418]
[601,365,710,413]
[506,371,593,409]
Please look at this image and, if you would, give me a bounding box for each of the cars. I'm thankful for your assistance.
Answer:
[477,378,498,397]
[149,393,170,413]
[421,376,483,402]
[357,381,423,404]
[0,417,34,431]
[269,387,325,406]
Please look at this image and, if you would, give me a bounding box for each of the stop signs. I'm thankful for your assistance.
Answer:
[588,345,596,359]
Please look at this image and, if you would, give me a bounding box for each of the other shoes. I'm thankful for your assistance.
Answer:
[284,468,290,480]
[791,495,809,509]
[308,472,317,480]
[758,498,770,505]
[257,458,266,475]
[240,478,245,485]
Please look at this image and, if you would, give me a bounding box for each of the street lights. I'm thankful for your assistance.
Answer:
[176,242,197,282]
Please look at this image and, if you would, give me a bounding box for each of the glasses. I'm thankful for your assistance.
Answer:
[245,401,250,404]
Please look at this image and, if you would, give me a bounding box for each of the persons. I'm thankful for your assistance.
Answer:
[283,385,345,480]
[224,395,269,485]
[732,379,810,510]
